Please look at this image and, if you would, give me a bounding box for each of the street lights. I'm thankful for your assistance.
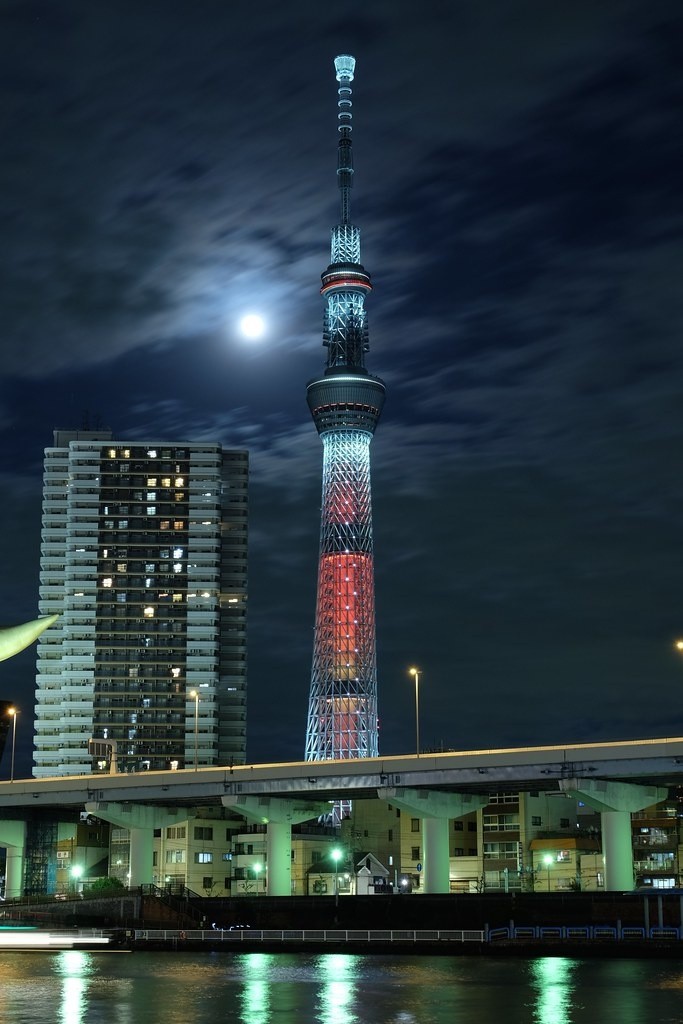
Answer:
[544,856,554,893]
[8,708,17,784]
[71,866,82,919]
[410,666,423,760]
[188,690,200,772]
[332,845,347,921]
